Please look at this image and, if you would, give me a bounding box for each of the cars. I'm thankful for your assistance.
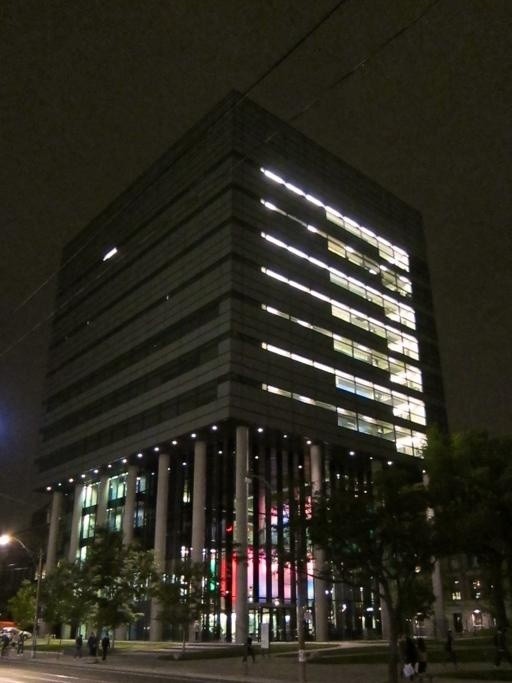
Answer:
[0,620,33,647]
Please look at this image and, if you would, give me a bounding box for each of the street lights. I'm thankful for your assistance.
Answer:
[239,470,277,503]
[0,534,43,659]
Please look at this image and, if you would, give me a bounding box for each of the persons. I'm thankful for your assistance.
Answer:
[102,632,110,661]
[396,633,428,683]
[492,629,505,669]
[441,630,458,669]
[0,631,24,657]
[73,635,82,661]
[86,632,98,663]
[242,634,255,663]
